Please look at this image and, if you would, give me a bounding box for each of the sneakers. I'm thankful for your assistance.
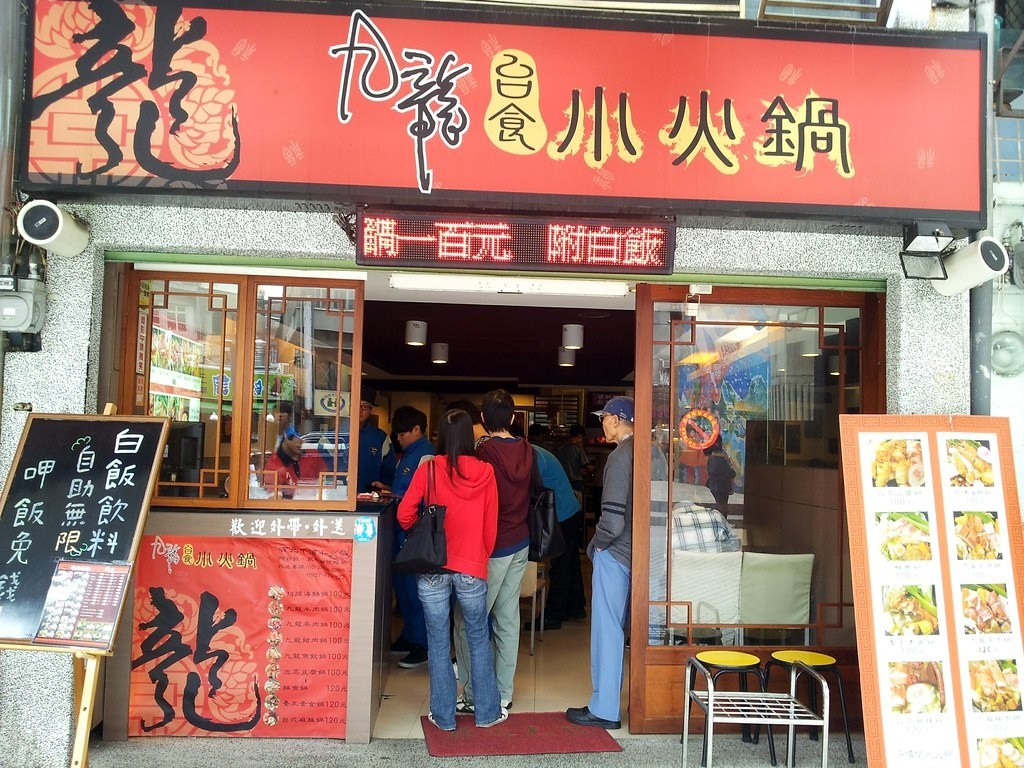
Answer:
[428,711,456,731]
[456,697,475,715]
[398,643,428,668]
[476,707,508,728]
[390,634,413,655]
[500,700,513,709]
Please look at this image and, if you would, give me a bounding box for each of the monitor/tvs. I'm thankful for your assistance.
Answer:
[156,421,205,498]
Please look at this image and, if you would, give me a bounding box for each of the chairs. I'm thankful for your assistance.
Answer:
[522,559,547,655]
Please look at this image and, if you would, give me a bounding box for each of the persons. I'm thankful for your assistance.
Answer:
[451,389,532,715]
[392,405,437,668]
[260,428,328,500]
[397,408,509,731]
[479,422,622,556]
[317,390,395,511]
[650,471,742,646]
[525,445,587,632]
[272,402,294,438]
[566,396,668,730]
[701,433,736,520]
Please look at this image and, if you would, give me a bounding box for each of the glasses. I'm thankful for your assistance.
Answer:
[599,414,620,423]
[360,405,373,410]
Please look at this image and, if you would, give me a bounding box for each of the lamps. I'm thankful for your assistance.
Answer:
[405,320,427,345]
[558,346,576,366]
[431,342,449,363]
[899,219,956,280]
[562,324,583,349]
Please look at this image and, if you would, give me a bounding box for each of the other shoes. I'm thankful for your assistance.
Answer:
[525,616,561,630]
[560,607,587,621]
[626,637,630,649]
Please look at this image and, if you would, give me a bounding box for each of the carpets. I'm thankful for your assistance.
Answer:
[421,712,624,756]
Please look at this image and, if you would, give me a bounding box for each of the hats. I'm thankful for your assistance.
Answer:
[361,386,378,407]
[591,396,634,424]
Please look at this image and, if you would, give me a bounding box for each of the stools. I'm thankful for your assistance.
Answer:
[753,650,855,768]
[679,650,777,768]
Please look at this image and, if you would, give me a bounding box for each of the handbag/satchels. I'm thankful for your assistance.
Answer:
[390,459,447,575]
[526,446,567,562]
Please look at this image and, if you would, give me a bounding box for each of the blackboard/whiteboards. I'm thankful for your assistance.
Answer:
[0,414,172,654]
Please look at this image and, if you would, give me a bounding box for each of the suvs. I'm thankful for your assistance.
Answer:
[250,426,350,486]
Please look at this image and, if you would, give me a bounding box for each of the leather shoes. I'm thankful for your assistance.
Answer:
[566,706,621,729]
